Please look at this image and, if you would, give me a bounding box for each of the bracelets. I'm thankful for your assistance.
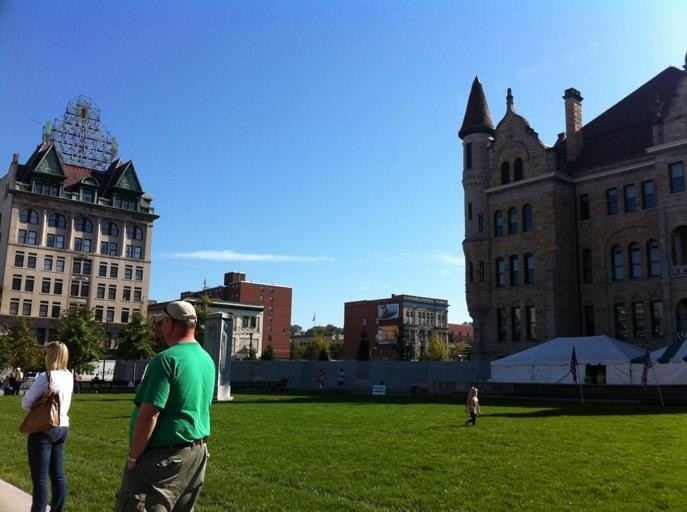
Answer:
[127,453,136,463]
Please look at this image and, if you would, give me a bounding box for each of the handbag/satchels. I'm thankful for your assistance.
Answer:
[19,392,60,433]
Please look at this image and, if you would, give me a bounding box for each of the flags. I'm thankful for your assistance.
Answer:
[569,347,578,382]
[641,351,654,392]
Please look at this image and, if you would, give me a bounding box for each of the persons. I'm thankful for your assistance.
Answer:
[21,340,76,512]
[8,373,15,387]
[95,374,99,380]
[337,367,345,387]
[13,367,24,395]
[463,383,482,426]
[114,300,218,512]
[318,369,328,392]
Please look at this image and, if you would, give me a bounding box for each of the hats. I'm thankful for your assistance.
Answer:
[155,301,198,322]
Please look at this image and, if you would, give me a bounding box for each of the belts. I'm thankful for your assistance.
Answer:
[158,439,205,448]
